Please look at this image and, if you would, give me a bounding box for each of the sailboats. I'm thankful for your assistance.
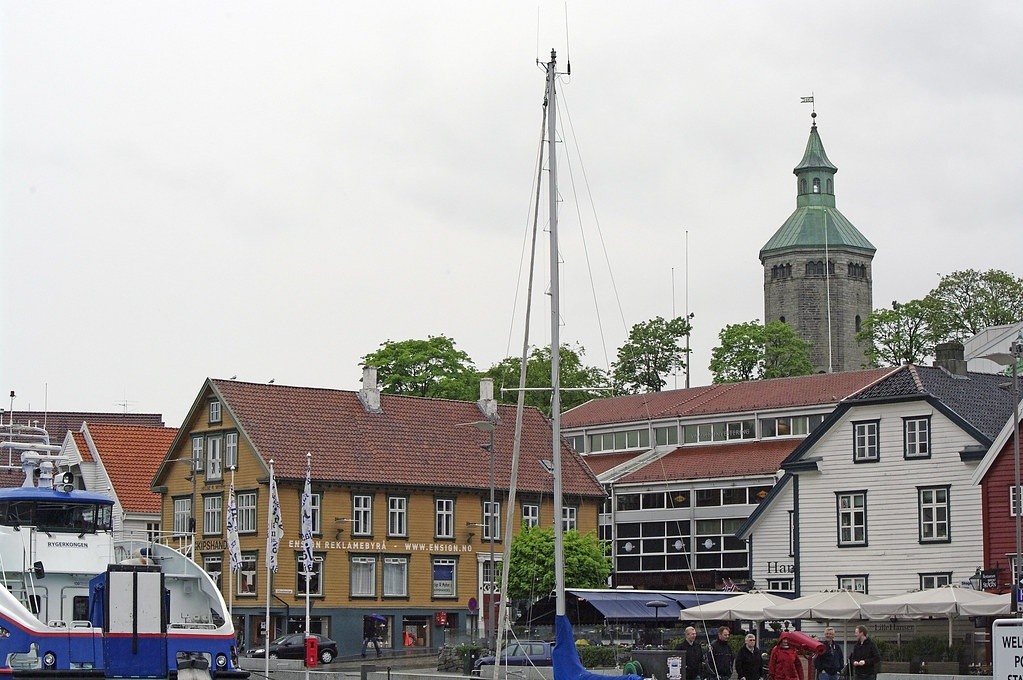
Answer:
[468,34,646,680]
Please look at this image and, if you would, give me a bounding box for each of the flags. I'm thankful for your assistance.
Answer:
[266,468,284,575]
[301,468,314,573]
[226,481,243,575]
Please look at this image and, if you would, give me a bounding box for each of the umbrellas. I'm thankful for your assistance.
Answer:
[368,612,387,623]
[678,588,793,647]
[862,586,1011,647]
[762,585,881,666]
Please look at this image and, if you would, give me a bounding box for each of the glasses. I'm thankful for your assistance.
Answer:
[750,640,755,641]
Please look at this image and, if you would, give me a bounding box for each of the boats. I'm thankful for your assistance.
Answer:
[0,381,254,680]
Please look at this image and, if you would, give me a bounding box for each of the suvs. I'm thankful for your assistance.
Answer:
[473,638,581,675]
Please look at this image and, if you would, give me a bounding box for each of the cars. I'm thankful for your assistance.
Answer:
[243,631,339,665]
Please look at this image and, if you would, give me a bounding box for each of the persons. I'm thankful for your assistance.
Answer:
[769,631,804,680]
[361,617,382,657]
[707,626,734,680]
[814,627,844,680]
[674,626,704,680]
[735,633,765,680]
[850,624,880,680]
